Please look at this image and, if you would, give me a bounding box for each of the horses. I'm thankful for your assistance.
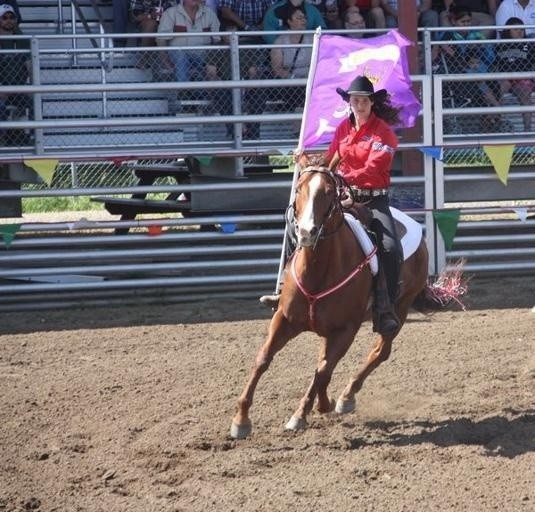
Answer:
[230,151,478,440]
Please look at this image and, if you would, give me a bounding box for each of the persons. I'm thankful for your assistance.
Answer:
[0,1,33,146]
[258,73,402,334]
[128,1,535,138]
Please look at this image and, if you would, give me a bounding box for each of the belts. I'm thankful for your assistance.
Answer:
[345,188,389,198]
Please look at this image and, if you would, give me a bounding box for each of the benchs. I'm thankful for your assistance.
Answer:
[160,68,535,140]
[90,197,221,234]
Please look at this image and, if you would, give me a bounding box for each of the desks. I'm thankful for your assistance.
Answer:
[123,164,288,232]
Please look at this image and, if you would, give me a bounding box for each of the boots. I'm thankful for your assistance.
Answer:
[377,250,403,333]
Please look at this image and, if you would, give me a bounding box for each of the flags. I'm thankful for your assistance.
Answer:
[301,30,421,154]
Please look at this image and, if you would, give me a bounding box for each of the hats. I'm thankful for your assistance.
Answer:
[337,75,386,102]
